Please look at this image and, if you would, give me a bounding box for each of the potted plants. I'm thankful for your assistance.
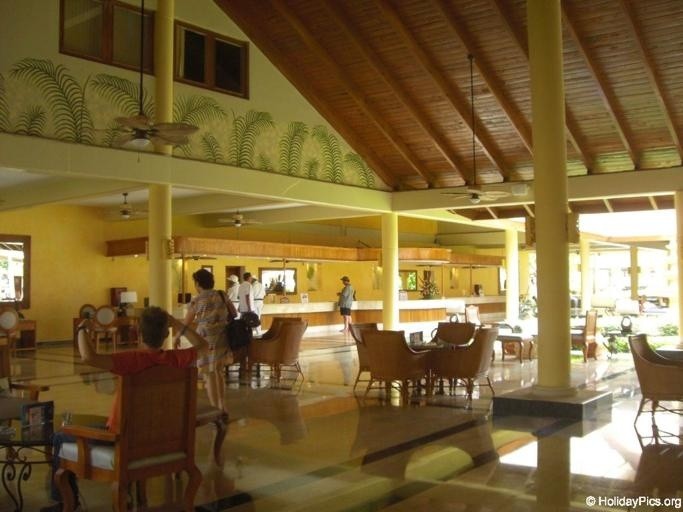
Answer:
[420,280,439,299]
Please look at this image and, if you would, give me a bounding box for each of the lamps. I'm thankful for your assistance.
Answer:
[130,138,151,147]
[235,224,241,226]
[469,198,481,204]
[120,292,137,309]
[122,215,130,218]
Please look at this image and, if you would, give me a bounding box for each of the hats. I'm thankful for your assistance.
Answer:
[341,277,349,281]
[227,275,238,283]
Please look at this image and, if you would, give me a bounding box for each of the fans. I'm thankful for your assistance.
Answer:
[94,0,201,148]
[218,211,262,223]
[105,192,149,215]
[439,54,510,201]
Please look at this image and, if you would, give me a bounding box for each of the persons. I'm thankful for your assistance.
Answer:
[173,268,237,411]
[237,272,255,313]
[41,306,208,512]
[336,276,354,333]
[226,274,241,320]
[251,275,266,336]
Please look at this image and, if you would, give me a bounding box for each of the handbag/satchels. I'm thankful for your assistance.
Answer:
[227,319,252,349]
[242,311,260,327]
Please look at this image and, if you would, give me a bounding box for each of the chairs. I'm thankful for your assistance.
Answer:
[0,304,683,512]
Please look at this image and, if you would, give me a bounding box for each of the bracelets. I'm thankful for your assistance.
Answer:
[77,326,85,331]
[181,325,188,335]
